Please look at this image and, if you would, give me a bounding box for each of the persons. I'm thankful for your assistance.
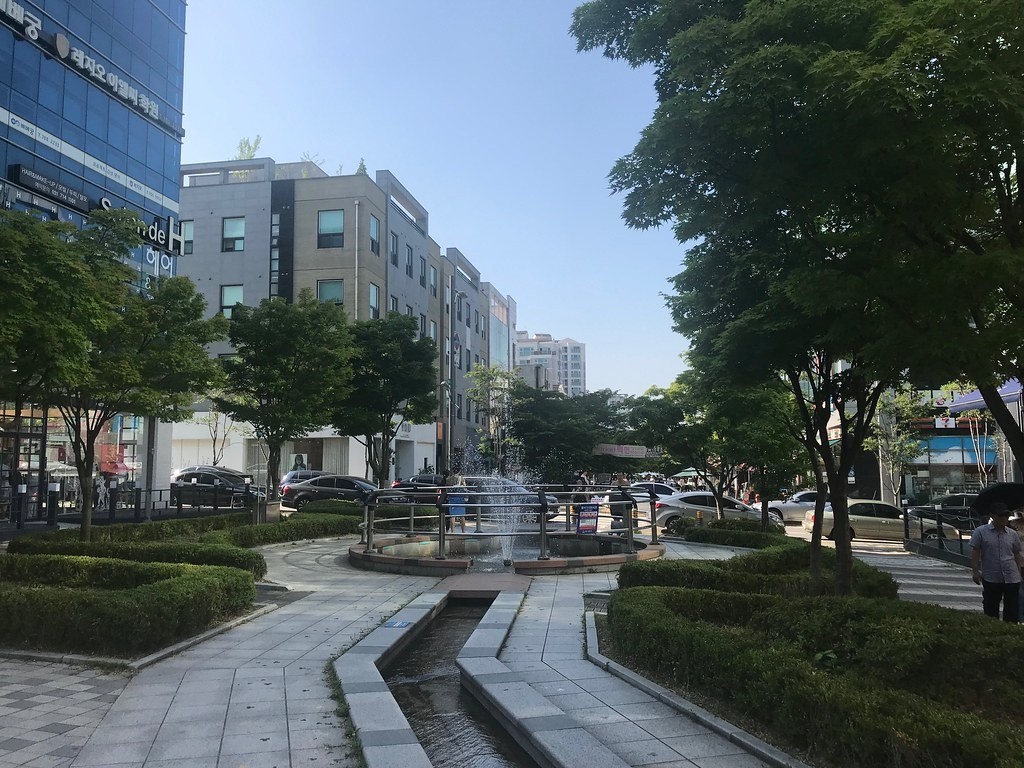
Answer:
[571,481,588,525]
[437,470,450,533]
[969,511,1024,624]
[292,455,306,471]
[445,466,467,533]
[1007,517,1024,624]
[640,474,707,492]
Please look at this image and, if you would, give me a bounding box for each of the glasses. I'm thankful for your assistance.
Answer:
[994,512,1010,517]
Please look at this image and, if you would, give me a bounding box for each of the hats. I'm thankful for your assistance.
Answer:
[990,502,1012,515]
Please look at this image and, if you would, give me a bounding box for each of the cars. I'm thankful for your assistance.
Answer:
[460,475,560,524]
[904,493,993,530]
[606,482,681,513]
[390,474,493,520]
[170,470,266,506]
[647,490,788,534]
[280,475,409,514]
[803,500,962,541]
[280,470,339,496]
[748,489,854,524]
[172,466,271,495]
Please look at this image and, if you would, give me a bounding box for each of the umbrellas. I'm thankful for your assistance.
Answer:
[674,467,712,490]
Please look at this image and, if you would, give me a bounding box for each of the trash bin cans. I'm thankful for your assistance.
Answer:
[251,501,280,525]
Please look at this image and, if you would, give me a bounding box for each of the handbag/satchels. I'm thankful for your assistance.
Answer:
[436,488,443,507]
